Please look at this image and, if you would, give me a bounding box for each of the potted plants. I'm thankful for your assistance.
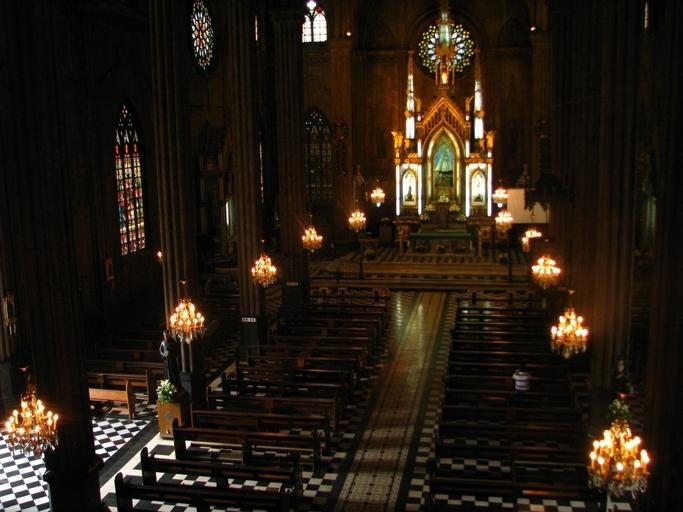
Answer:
[156,380,189,439]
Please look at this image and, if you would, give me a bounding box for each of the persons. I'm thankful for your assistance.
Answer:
[160,330,182,391]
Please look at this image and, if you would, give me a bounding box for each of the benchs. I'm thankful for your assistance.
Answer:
[428,293,595,510]
[89,380,137,423]
[114,403,333,512]
[87,367,150,405]
[206,293,392,434]
[95,328,165,376]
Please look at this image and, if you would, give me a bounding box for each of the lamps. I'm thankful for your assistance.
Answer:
[370,186,385,208]
[5,384,59,457]
[251,255,277,289]
[348,211,366,233]
[302,227,323,253]
[522,227,542,252]
[492,186,508,208]
[495,211,513,233]
[169,298,205,345]
[586,418,651,500]
[531,254,561,289]
[551,306,589,359]
